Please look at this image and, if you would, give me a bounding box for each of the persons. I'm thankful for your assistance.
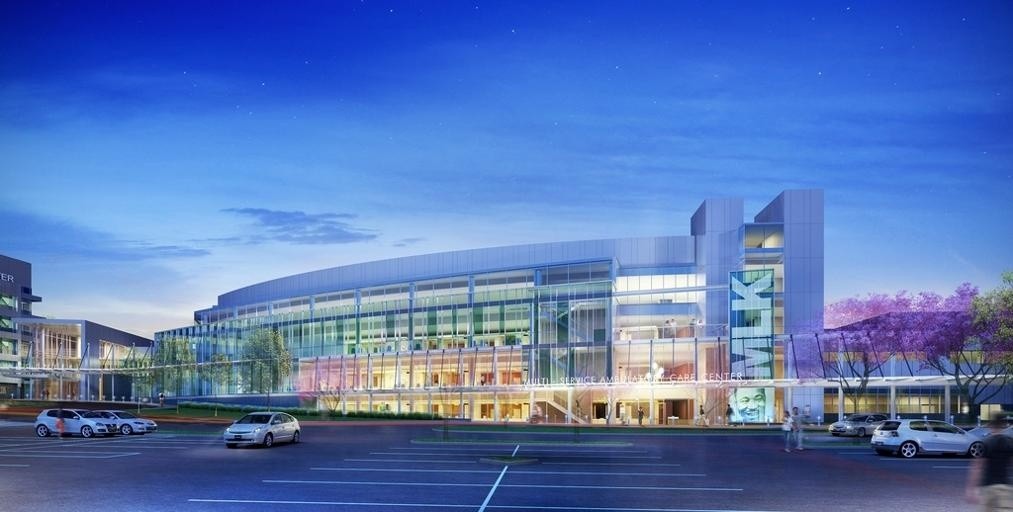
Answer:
[159,394,164,407]
[725,403,734,425]
[963,409,1013,512]
[56,406,66,440]
[689,318,704,337]
[664,318,677,337]
[781,407,804,453]
[637,406,645,425]
[699,405,706,427]
[734,386,767,424]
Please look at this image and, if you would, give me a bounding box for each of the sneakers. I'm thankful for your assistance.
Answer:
[784,446,804,455]
